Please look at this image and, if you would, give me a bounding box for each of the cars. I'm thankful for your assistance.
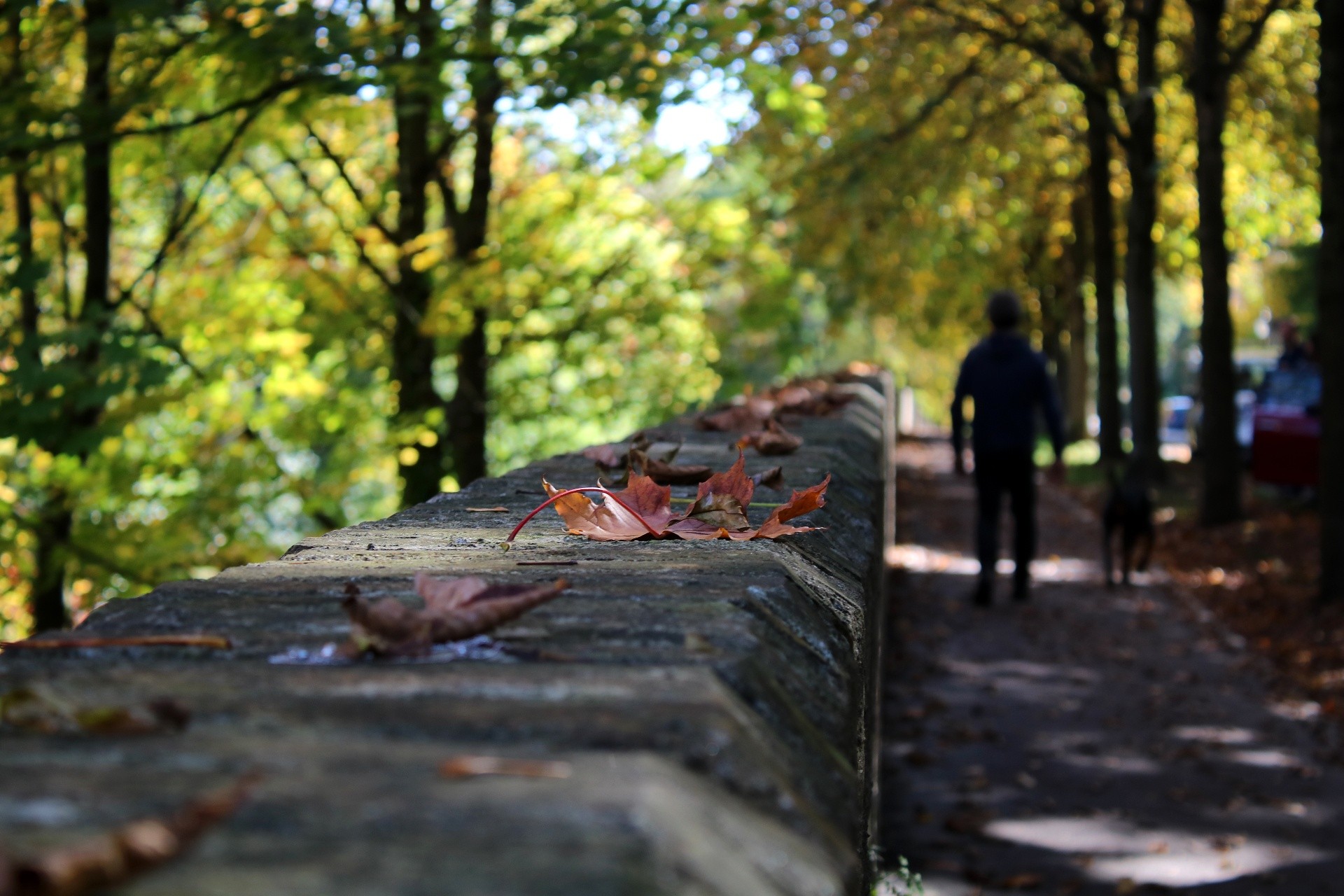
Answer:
[1249,345,1328,492]
[1152,355,1277,444]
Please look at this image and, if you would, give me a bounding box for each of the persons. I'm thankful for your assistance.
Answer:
[950,292,1068,610]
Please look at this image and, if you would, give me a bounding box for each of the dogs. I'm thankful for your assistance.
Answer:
[1094,469,1156,594]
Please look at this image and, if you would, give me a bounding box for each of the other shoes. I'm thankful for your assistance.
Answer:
[1014,574,1029,600]
[973,576,992,607]
